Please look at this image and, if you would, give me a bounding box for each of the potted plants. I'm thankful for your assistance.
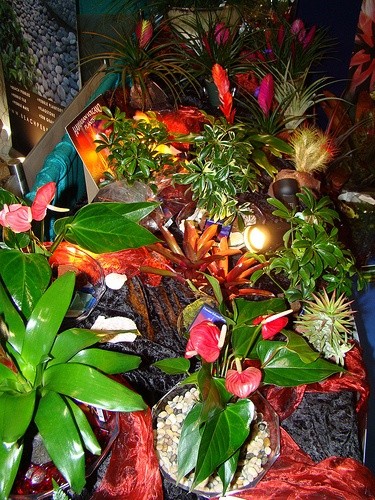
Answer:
[1,0,375,500]
